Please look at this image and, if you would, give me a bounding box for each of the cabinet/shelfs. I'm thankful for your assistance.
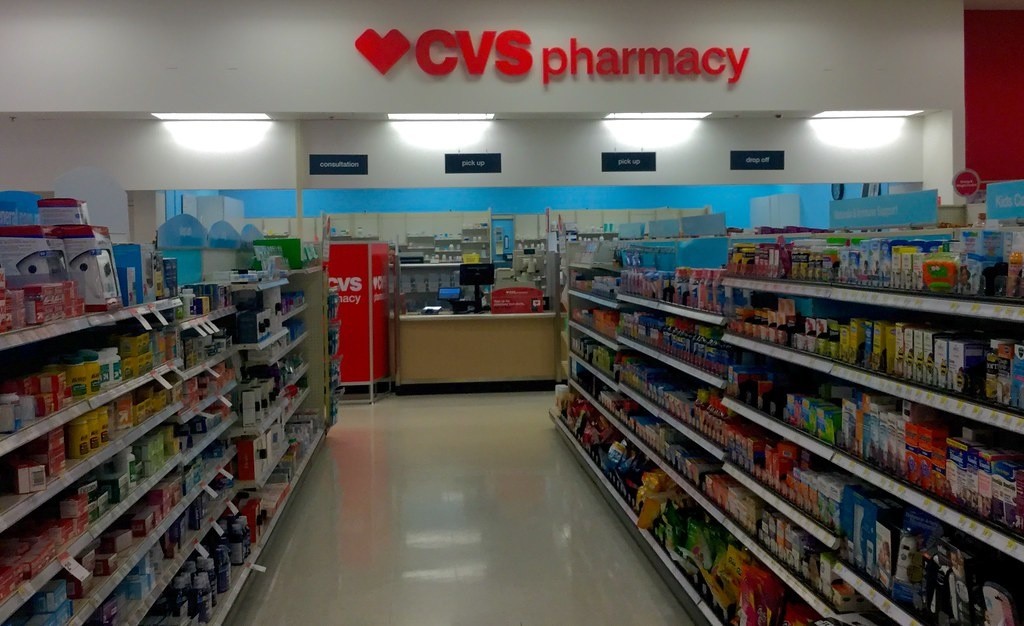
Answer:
[548,284,624,511]
[610,240,729,617]
[203,249,328,547]
[389,222,620,268]
[0,246,262,626]
[697,228,1024,626]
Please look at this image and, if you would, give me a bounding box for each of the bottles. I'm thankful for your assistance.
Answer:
[482,243,486,257]
[214,544,231,594]
[228,515,251,566]
[638,311,736,380]
[170,556,217,623]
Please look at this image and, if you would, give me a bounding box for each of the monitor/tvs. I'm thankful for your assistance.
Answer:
[459,264,494,285]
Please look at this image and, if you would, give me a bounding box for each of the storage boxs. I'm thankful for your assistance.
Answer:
[0,198,320,626]
[620,229,1024,626]
